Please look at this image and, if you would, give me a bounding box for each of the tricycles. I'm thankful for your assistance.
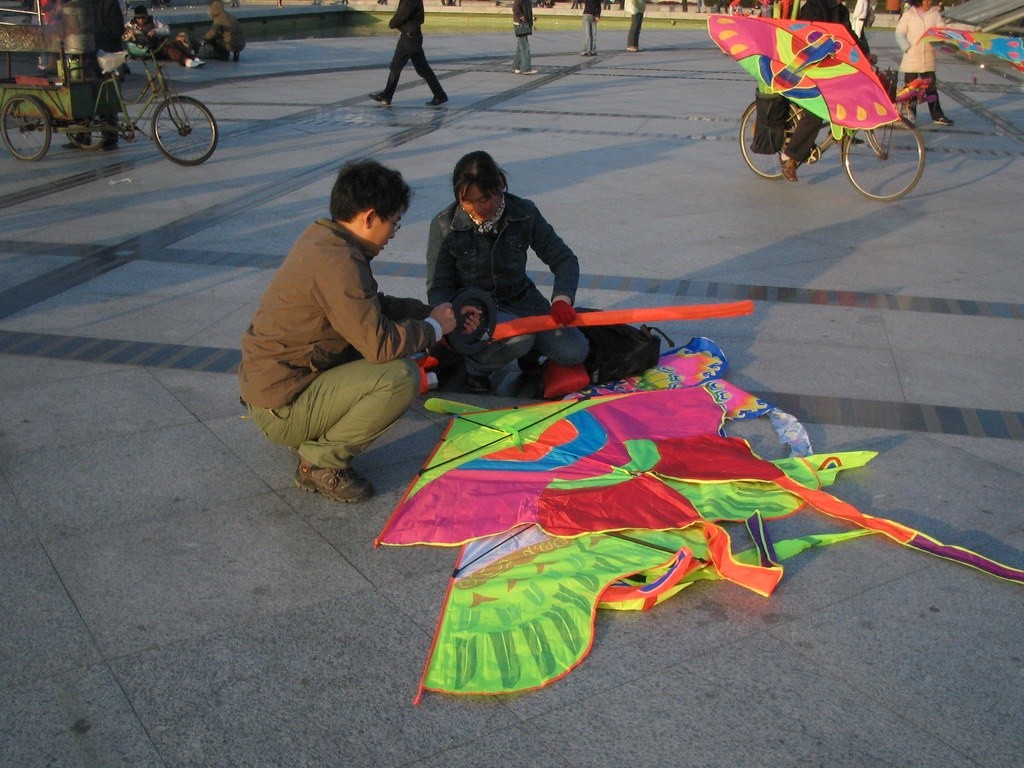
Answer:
[0,38,219,167]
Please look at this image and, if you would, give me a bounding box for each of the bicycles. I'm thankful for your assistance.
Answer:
[738,59,927,202]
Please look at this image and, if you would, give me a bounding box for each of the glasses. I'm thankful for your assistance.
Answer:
[382,215,402,232]
[136,15,147,20]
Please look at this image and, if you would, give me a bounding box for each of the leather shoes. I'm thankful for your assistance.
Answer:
[828,130,864,144]
[779,152,798,182]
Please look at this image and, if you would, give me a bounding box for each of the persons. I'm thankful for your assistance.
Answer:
[425,151,588,394]
[895,0,955,125]
[617,0,626,11]
[312,0,322,5]
[369,0,449,106]
[936,1,947,12]
[851,0,870,54]
[341,0,349,5]
[122,6,205,68]
[580,0,601,56]
[20,0,37,24]
[532,0,556,8]
[278,0,284,9]
[627,0,646,52]
[695,0,770,17]
[510,0,537,75]
[177,25,202,57]
[39,0,129,152]
[777,0,869,180]
[570,0,584,9]
[440,0,462,6]
[604,0,613,10]
[200,0,247,62]
[239,156,482,503]
[376,0,388,5]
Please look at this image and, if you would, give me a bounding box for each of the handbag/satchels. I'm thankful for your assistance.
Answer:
[515,21,532,36]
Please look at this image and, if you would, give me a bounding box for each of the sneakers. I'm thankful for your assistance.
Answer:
[294,456,373,502]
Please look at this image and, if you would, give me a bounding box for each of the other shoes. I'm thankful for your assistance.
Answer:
[232,52,239,62]
[512,68,521,73]
[517,350,539,373]
[626,46,642,52]
[61,136,92,149]
[194,57,206,65]
[185,59,198,67]
[523,70,537,74]
[369,92,392,105]
[933,117,953,125]
[98,141,118,151]
[425,92,448,106]
[461,364,491,392]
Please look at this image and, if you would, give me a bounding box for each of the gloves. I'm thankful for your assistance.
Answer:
[552,300,576,329]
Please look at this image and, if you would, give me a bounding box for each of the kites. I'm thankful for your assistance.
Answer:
[374,27,917,158]
[707,13,937,140]
[920,25,1024,72]
[374,289,1024,706]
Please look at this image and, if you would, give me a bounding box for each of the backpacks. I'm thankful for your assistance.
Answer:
[573,307,674,385]
